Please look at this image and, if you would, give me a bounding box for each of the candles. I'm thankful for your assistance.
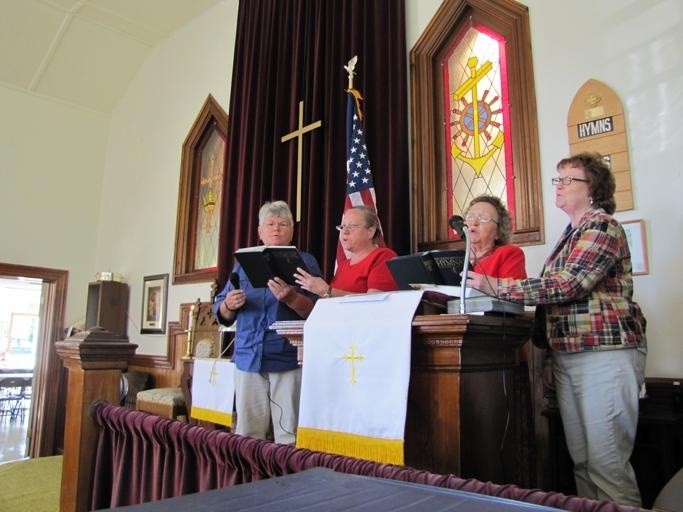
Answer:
[188,304,195,329]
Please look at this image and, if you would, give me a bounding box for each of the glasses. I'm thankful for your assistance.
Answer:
[464,212,502,226]
[335,223,370,231]
[551,176,591,186]
[262,220,294,229]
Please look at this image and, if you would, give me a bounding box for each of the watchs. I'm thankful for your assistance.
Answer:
[324,286,332,298]
[224,300,236,312]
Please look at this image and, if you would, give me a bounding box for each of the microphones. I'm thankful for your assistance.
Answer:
[447,214,466,239]
[228,272,242,290]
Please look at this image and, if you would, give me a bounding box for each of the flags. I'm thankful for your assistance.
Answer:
[334,94,386,279]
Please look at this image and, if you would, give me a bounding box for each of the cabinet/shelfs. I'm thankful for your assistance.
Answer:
[86,280,130,339]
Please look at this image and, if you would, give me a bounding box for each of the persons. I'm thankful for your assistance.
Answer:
[463,196,538,485]
[212,201,323,446]
[461,153,648,508]
[294,206,398,298]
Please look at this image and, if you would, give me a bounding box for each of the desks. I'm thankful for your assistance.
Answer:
[97,466,566,512]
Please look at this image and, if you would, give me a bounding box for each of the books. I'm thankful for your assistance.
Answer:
[385,248,475,290]
[234,245,311,289]
[422,284,488,304]
[440,311,492,316]
[446,297,525,317]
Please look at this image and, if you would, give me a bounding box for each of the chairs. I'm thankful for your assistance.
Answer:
[136,297,221,424]
[11,378,33,420]
[0,377,24,422]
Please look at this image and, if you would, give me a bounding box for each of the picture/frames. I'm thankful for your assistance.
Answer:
[621,218,651,276]
[140,274,169,335]
